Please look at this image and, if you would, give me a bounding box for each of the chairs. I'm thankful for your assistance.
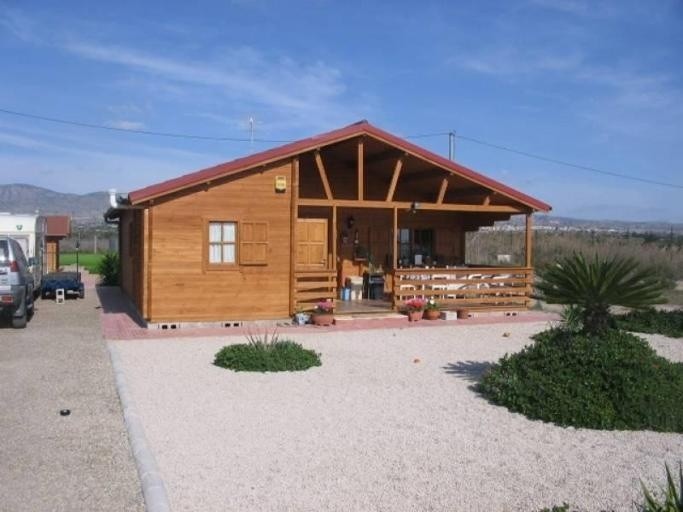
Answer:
[399,264,513,306]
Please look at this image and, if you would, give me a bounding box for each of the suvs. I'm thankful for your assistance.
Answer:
[0,237,35,328]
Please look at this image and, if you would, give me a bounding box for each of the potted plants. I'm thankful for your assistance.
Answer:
[293,305,305,326]
[424,300,441,320]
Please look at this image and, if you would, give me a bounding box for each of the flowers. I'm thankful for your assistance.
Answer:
[312,301,332,314]
[403,298,426,309]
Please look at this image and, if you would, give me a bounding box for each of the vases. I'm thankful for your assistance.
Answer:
[311,313,333,327]
[407,310,423,322]
